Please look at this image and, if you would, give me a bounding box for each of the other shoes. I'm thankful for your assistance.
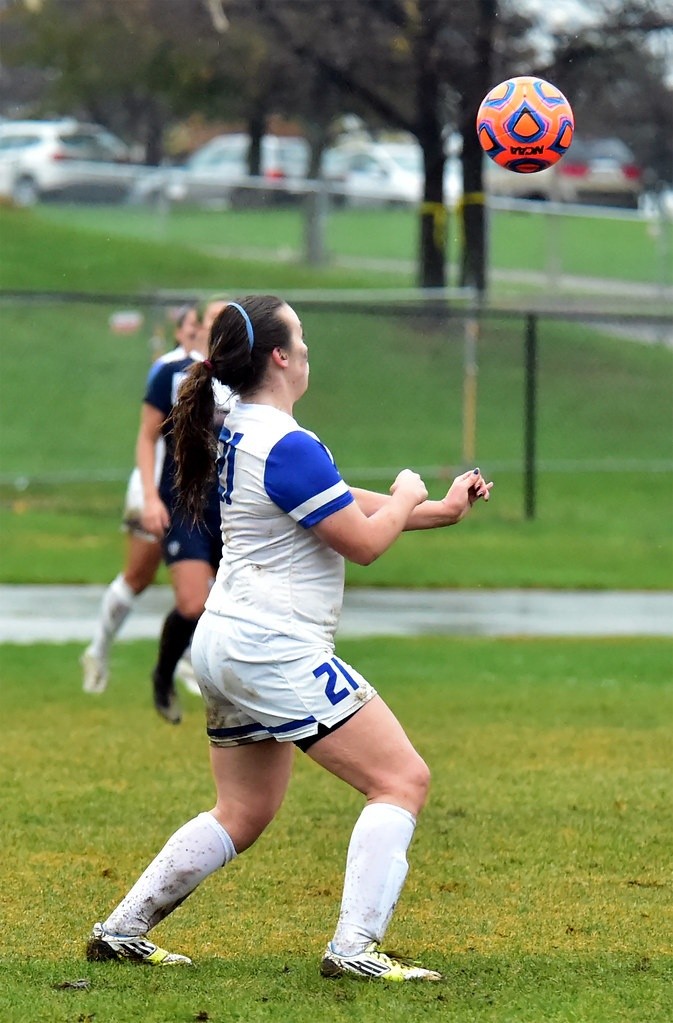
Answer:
[84,648,109,692]
[154,669,183,723]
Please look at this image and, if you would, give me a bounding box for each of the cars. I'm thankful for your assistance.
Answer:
[320,139,462,204]
[0,119,143,210]
[145,130,344,210]
[484,133,641,211]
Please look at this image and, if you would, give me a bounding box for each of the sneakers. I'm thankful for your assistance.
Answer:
[87,923,192,966]
[321,941,442,983]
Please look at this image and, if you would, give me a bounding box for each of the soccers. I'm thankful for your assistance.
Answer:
[476,75,576,174]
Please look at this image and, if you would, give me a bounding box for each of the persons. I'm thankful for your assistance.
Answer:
[83,305,215,697]
[84,295,494,984]
[137,294,225,725]
